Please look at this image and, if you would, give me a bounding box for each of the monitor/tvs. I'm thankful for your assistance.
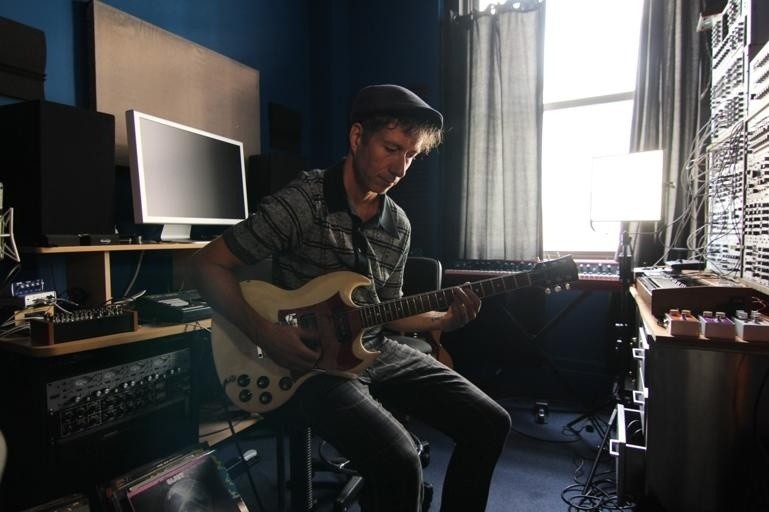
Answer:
[124,109,250,243]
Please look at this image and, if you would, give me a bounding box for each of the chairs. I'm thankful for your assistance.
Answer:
[318,256,444,512]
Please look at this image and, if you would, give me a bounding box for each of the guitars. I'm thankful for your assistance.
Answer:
[210,254,580,415]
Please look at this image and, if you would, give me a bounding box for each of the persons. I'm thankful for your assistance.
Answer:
[190,84,511,512]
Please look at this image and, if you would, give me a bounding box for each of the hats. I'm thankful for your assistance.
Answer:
[349,83,443,128]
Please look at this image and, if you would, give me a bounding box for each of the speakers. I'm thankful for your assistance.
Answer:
[248,153,303,209]
[0,97,121,246]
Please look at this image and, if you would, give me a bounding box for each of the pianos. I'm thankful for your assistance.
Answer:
[632,262,755,324]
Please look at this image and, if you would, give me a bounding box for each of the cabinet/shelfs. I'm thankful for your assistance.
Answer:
[610,305,767,511]
[3,238,268,511]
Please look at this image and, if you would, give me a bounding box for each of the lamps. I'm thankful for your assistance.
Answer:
[566,149,668,435]
